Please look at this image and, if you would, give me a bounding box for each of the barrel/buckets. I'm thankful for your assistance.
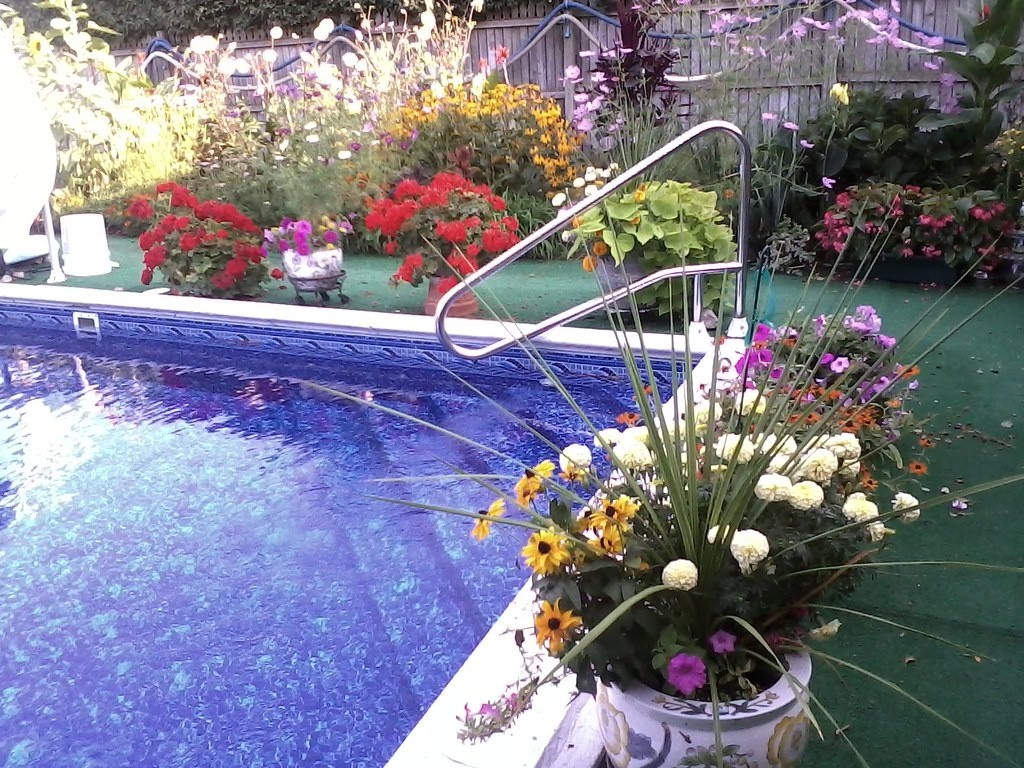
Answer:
[60,213,120,277]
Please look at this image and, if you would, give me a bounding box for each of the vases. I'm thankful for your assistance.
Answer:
[862,251,968,288]
[597,641,813,768]
[425,273,481,316]
[594,255,664,316]
[279,247,348,308]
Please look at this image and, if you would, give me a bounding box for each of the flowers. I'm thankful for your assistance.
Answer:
[816,179,1014,274]
[555,112,736,316]
[407,235,1024,768]
[364,175,522,293]
[127,178,265,294]
[266,214,355,255]
